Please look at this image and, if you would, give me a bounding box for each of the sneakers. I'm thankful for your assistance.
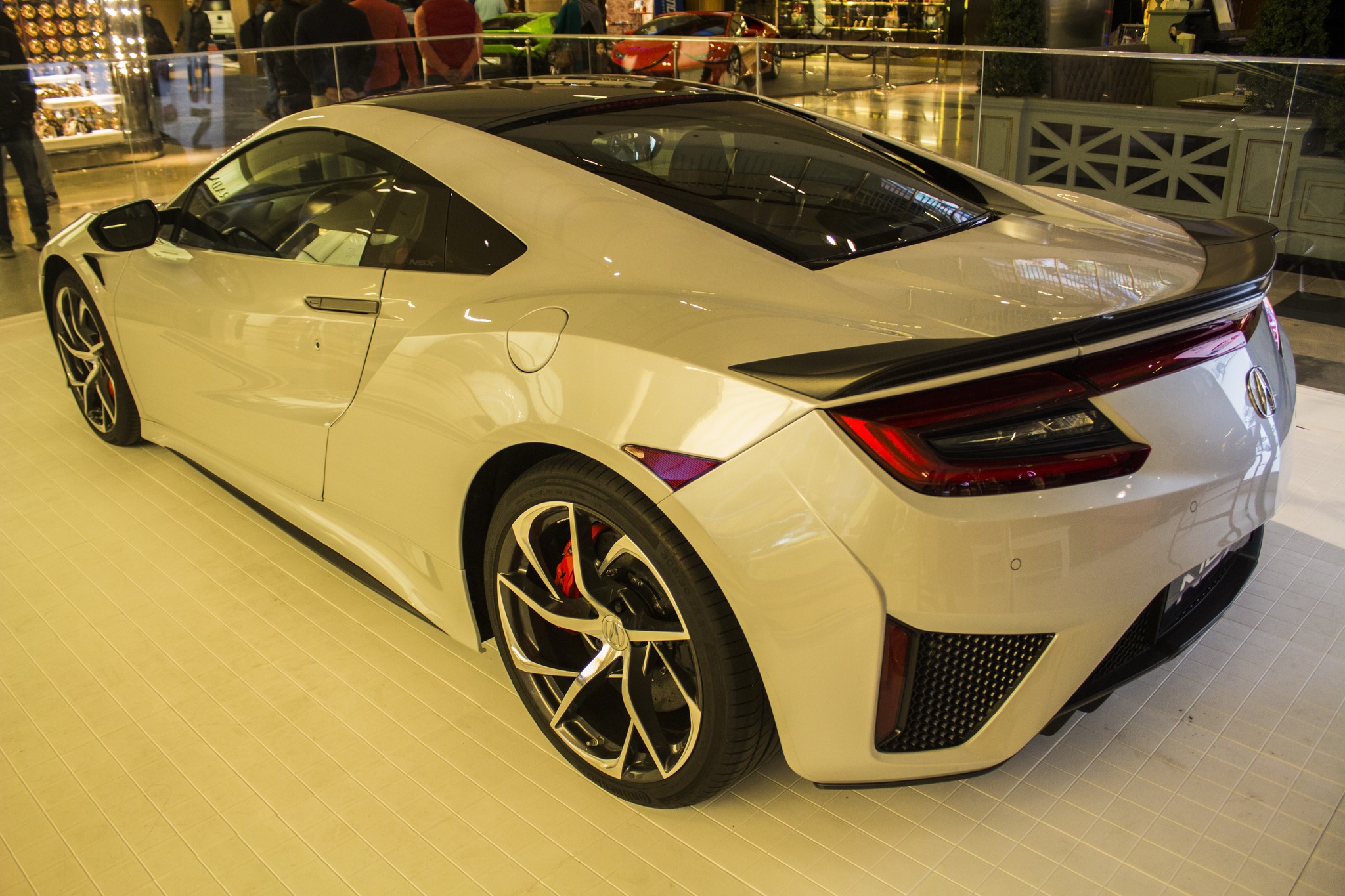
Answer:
[0,241,16,257]
[45,193,59,205]
[30,226,52,249]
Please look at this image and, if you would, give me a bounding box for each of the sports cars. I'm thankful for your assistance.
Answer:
[610,11,785,96]
[32,72,1296,812]
[477,12,568,76]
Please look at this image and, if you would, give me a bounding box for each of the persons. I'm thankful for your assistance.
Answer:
[256,0,612,121]
[140,0,216,97]
[0,0,61,258]
